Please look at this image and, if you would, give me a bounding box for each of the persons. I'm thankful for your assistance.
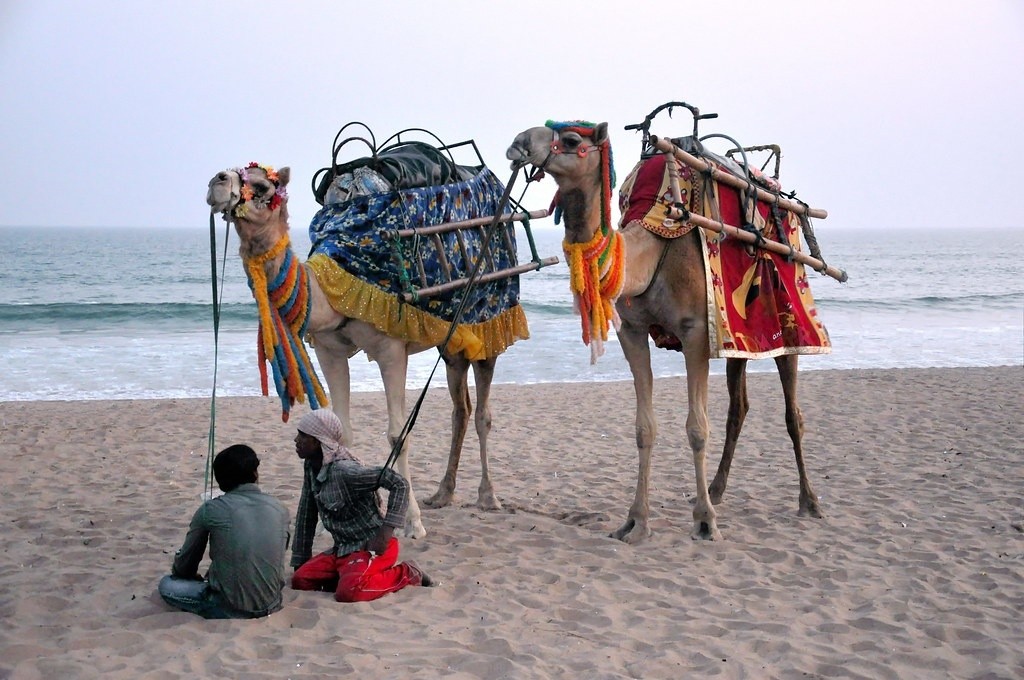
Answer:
[157,444,290,619]
[290,410,434,602]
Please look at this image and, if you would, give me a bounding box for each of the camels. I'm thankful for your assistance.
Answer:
[506,118,826,545]
[207,163,521,538]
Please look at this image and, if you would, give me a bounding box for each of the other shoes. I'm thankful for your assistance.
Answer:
[409,559,436,587]
